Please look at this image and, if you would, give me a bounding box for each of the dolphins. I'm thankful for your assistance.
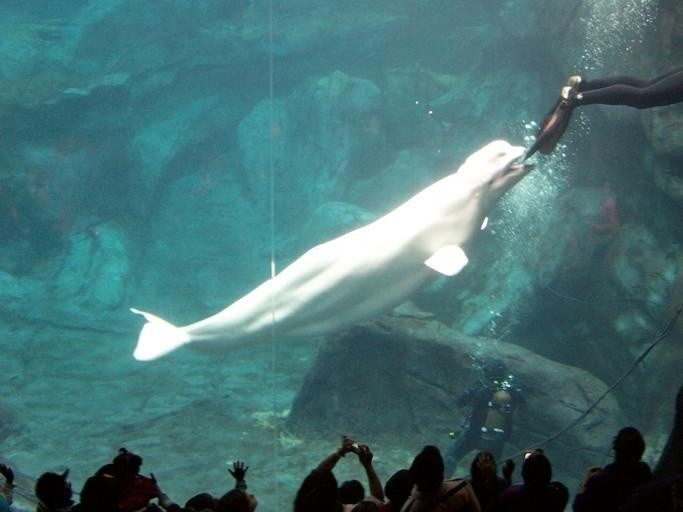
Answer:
[128,138,536,363]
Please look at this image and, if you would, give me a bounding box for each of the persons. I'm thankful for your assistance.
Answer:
[1,447,258,512]
[293,386,682,512]
[521,66,683,162]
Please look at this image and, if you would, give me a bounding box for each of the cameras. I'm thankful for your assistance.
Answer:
[351,442,363,456]
